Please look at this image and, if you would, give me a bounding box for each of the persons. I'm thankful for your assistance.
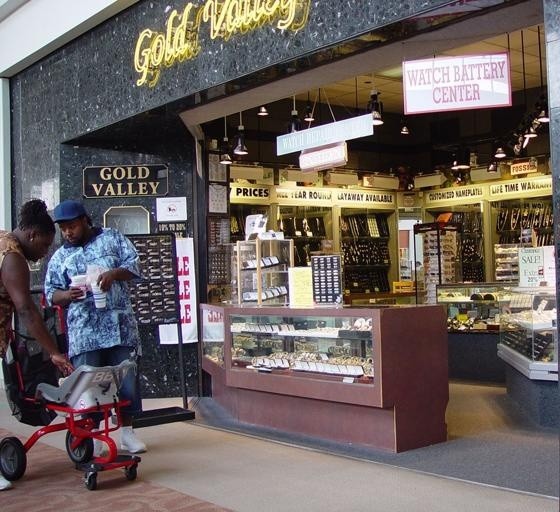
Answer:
[42,200,150,454]
[0,196,78,381]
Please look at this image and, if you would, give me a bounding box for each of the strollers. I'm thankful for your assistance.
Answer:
[0,289,142,492]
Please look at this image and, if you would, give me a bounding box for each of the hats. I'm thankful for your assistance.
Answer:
[53,200,84,224]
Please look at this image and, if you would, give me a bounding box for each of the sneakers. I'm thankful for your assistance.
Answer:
[0,478,11,492]
[91,428,104,455]
[120,429,146,453]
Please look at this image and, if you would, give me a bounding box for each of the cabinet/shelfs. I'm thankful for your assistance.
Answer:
[494,290,559,428]
[230,176,560,301]
[199,307,451,453]
[436,282,514,386]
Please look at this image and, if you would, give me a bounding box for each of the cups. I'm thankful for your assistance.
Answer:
[92,283,107,309]
[75,283,86,300]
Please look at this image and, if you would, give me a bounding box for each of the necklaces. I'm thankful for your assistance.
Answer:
[492,198,555,247]
[340,214,390,241]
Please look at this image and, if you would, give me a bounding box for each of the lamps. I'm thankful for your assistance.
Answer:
[235,110,248,156]
[399,121,411,136]
[303,90,316,121]
[256,105,274,118]
[214,118,235,167]
[491,27,548,162]
[367,77,386,125]
